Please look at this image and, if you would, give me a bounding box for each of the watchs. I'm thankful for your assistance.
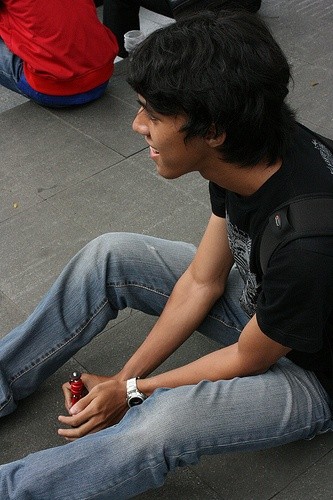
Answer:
[125,378,148,409]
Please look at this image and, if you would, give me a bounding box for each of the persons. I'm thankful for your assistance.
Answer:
[94,0,269,61]
[0,11,333,500]
[0,0,120,109]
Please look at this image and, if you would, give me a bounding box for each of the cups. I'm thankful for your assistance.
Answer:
[124,30,144,61]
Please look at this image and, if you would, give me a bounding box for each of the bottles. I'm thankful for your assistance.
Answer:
[70,371,87,406]
